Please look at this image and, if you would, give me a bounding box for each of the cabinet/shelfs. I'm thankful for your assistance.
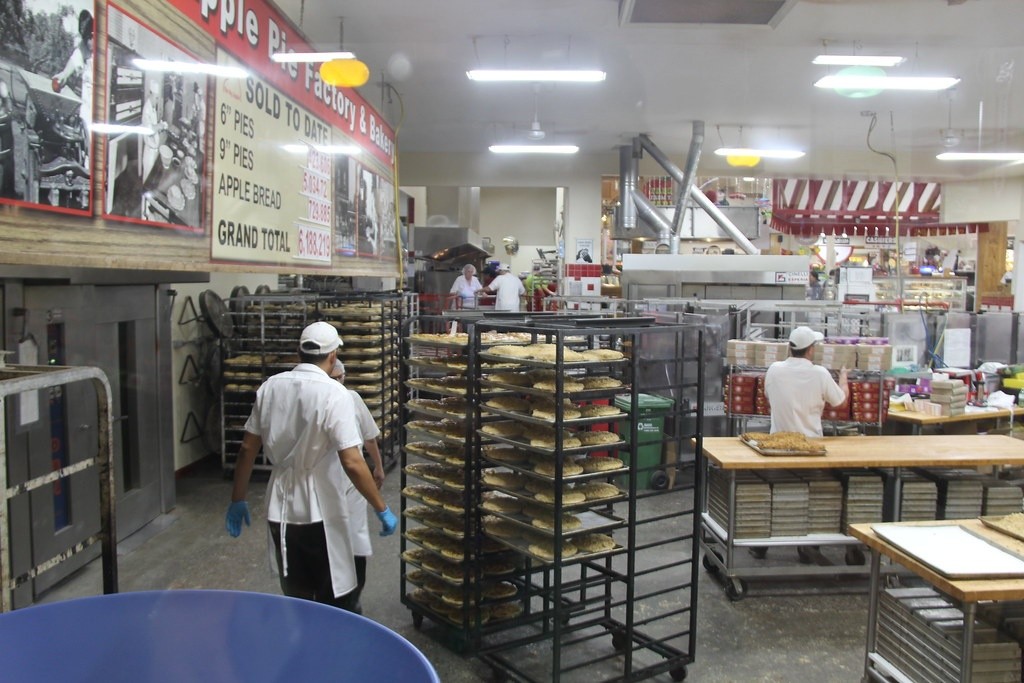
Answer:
[392,310,715,683]
[219,291,398,485]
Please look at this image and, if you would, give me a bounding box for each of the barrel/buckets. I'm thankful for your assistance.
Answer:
[418,294,462,331]
[575,398,617,459]
[0,589,441,683]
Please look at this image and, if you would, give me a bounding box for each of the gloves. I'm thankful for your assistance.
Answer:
[224,501,251,536]
[374,505,397,537]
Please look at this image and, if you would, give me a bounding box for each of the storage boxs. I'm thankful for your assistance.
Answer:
[713,335,1019,430]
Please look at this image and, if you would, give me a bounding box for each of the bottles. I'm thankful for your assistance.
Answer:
[824,276,838,300]
[601,273,619,286]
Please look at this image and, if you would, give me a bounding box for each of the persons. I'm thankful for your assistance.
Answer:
[224,319,398,615]
[475,262,527,310]
[52,8,94,156]
[141,80,164,185]
[810,270,822,300]
[447,264,488,311]
[382,201,396,239]
[330,357,385,597]
[748,327,854,563]
[542,279,558,296]
[521,271,545,311]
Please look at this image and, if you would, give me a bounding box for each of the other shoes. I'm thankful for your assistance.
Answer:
[748,547,765,559]
[801,552,825,562]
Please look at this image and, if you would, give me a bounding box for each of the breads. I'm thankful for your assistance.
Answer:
[481,342,626,564]
[405,332,584,629]
[221,302,400,443]
[742,430,826,451]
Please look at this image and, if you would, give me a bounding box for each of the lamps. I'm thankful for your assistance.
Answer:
[458,34,611,89]
[485,121,588,154]
[811,41,959,92]
[525,101,547,141]
[811,32,905,66]
[939,104,961,147]
[271,3,357,63]
[935,100,1023,163]
[707,122,813,158]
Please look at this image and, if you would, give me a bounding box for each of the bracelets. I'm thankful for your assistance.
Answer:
[230,497,246,502]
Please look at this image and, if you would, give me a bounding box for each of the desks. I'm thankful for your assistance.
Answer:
[887,405,1024,436]
[849,514,1024,680]
[694,435,1024,604]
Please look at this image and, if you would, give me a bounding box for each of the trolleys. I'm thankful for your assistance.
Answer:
[221,287,418,481]
[399,309,707,682]
[454,291,497,328]
[699,455,900,602]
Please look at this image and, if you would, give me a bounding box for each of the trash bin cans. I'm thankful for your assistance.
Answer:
[614,392,676,494]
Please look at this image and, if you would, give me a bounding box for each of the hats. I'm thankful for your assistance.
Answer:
[811,271,821,281]
[498,262,511,270]
[300,321,344,355]
[328,359,346,377]
[789,327,824,350]
